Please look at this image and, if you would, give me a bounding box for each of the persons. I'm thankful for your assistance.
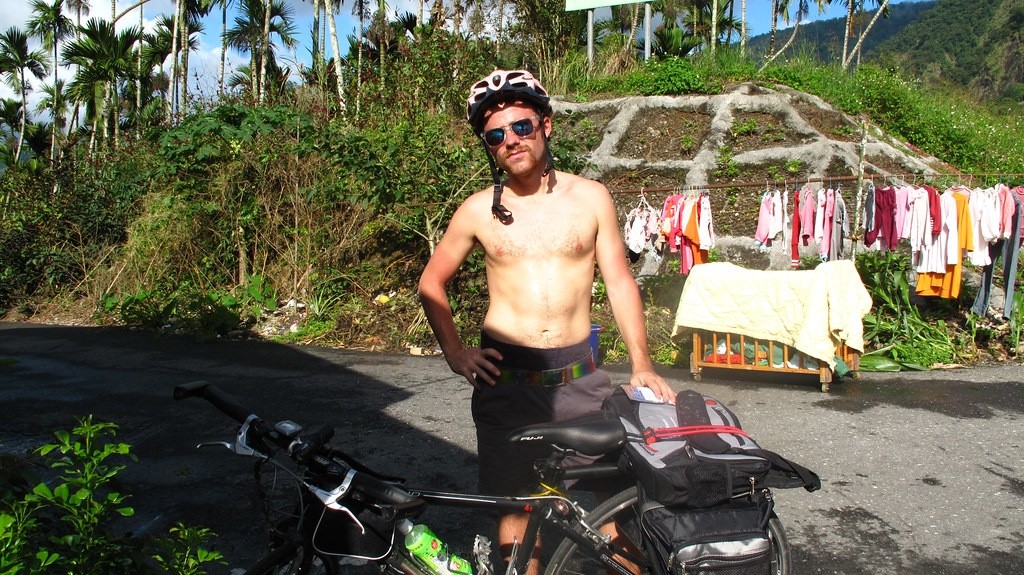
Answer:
[419,70,677,575]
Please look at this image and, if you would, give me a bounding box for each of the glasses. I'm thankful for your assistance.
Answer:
[480,113,542,149]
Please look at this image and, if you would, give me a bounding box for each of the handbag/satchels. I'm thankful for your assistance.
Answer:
[600,384,822,511]
[632,486,774,575]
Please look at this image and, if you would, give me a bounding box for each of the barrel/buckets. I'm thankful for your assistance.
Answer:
[590,325,605,368]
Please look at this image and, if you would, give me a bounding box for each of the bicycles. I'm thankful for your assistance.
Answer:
[174,381,790,575]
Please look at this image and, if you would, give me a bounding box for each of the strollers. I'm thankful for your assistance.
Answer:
[690,261,860,393]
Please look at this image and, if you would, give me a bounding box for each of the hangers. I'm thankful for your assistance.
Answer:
[621,172,1024,219]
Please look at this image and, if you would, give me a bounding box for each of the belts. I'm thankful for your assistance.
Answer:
[477,352,596,388]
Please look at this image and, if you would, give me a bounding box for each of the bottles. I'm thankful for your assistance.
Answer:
[397,518,472,575]
[752,361,768,366]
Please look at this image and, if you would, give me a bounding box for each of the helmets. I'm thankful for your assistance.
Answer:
[466,69,553,134]
